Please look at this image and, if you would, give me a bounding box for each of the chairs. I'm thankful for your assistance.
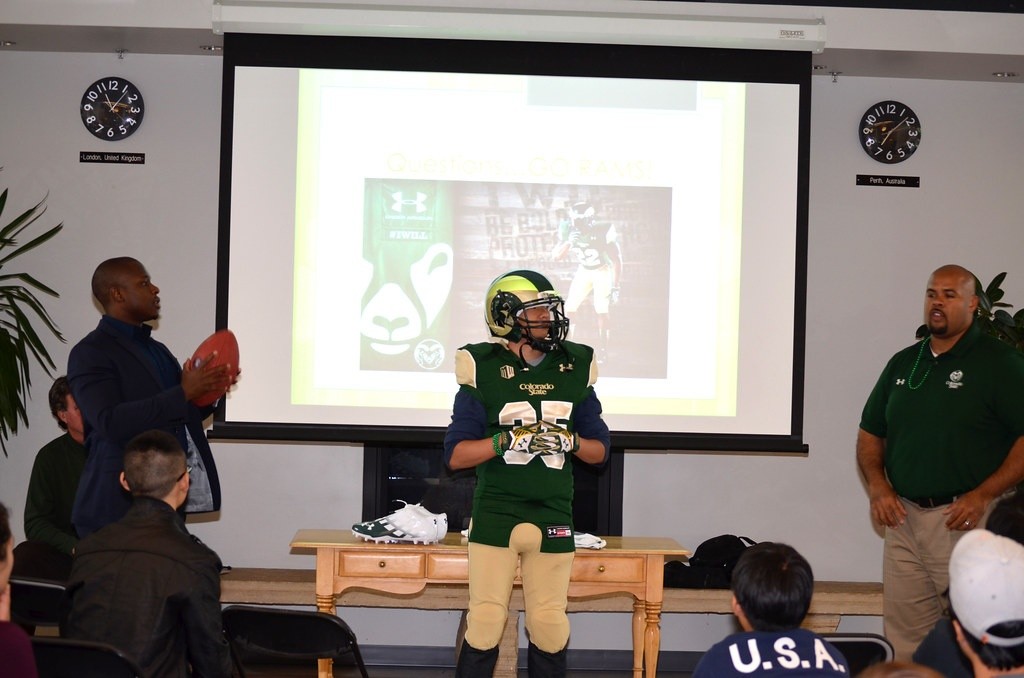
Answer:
[4,575,368,677]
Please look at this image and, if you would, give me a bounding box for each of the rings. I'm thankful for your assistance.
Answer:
[965,520,971,526]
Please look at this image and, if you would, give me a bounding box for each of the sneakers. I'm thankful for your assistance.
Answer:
[350,500,438,546]
[413,503,448,541]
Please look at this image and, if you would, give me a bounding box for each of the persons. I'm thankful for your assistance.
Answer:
[442,271,611,678]
[0,504,39,678]
[56,430,245,678]
[855,264,1024,658]
[858,660,944,678]
[24,375,86,579]
[912,494,1024,678]
[689,539,852,678]
[67,258,241,546]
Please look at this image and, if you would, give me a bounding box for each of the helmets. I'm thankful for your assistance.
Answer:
[483,268,571,354]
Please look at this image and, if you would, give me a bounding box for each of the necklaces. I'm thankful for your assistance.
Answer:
[908,334,937,389]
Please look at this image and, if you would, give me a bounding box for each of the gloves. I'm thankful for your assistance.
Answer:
[529,420,581,456]
[499,421,555,457]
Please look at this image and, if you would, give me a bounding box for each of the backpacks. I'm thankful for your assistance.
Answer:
[688,534,758,573]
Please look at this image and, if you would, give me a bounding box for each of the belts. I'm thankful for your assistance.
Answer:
[896,490,970,509]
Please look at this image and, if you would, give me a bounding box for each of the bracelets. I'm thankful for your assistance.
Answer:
[492,433,505,457]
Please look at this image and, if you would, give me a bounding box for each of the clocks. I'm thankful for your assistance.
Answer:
[860,100,922,163]
[81,76,145,143]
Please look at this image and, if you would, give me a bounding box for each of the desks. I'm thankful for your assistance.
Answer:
[291,527,689,678]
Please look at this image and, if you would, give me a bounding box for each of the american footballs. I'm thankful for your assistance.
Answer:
[190,330,240,406]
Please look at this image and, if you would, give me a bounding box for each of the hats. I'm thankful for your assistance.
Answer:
[946,528,1024,647]
